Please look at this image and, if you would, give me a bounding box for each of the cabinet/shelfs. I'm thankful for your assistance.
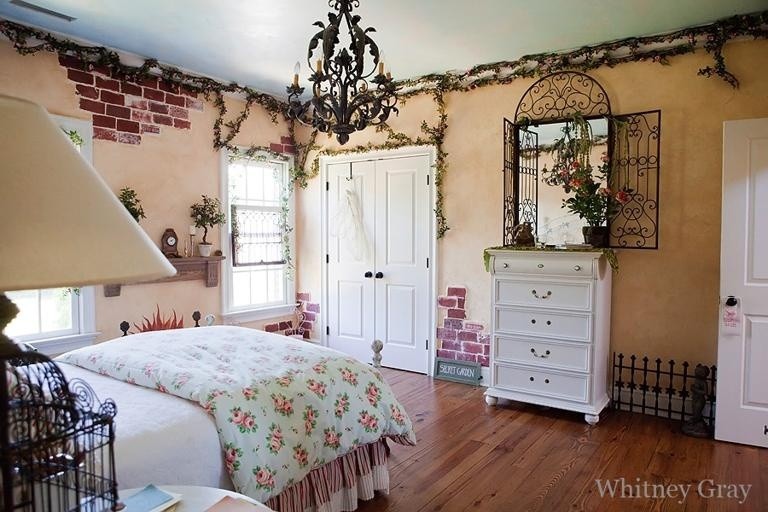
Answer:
[482,249,612,425]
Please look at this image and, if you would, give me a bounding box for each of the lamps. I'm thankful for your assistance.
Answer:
[0,91,178,512]
[284,0,403,146]
[541,120,581,194]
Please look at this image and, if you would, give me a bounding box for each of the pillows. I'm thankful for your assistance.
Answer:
[7,362,99,487]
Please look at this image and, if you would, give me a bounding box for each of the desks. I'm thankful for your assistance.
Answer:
[79,485,276,512]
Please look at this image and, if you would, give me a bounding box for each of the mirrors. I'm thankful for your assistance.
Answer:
[514,114,610,250]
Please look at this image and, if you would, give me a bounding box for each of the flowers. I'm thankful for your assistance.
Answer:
[190,195,227,245]
[540,109,629,227]
[560,161,594,226]
[573,156,629,226]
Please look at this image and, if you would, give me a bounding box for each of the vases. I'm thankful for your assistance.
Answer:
[198,245,213,256]
[591,227,609,248]
[583,227,592,243]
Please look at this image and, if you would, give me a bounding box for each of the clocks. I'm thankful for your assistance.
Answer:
[161,228,180,258]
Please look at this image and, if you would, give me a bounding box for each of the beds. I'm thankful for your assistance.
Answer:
[13,325,417,512]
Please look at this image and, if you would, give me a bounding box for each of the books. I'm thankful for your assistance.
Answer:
[122,482,182,512]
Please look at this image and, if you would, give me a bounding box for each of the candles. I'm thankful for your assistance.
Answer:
[189,225,196,235]
